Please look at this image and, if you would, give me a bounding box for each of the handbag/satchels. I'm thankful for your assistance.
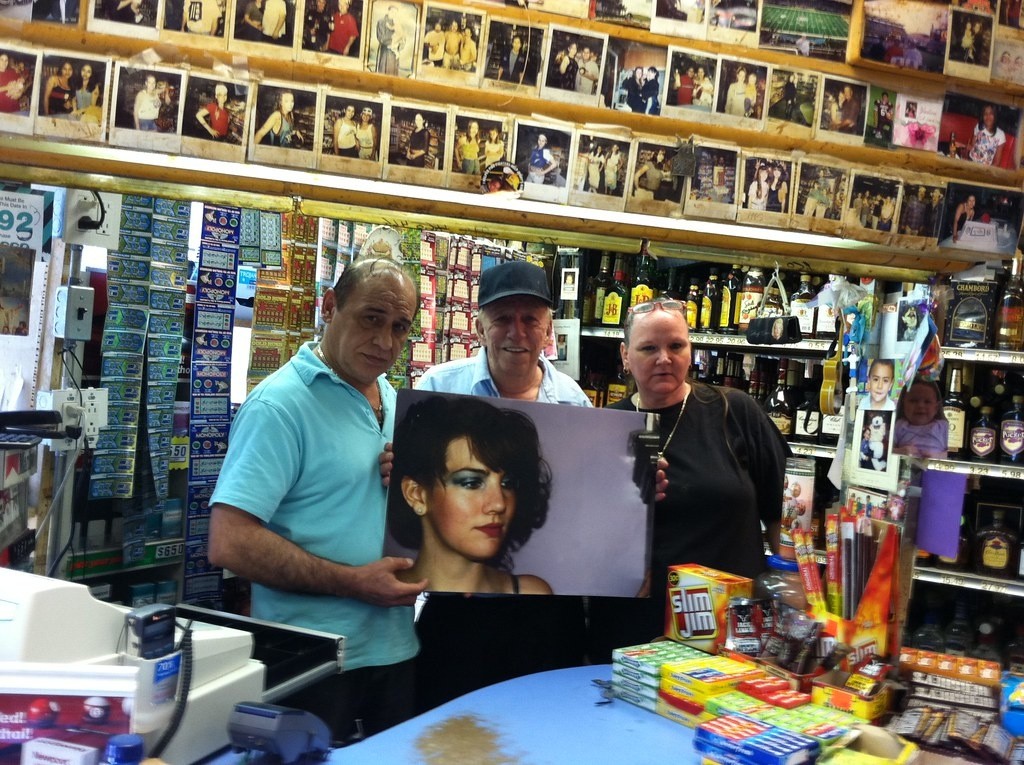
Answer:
[746,277,803,344]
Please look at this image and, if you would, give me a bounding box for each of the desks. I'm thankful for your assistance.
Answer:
[321,662,1024,765]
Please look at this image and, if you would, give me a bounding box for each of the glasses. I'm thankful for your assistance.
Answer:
[625,300,687,327]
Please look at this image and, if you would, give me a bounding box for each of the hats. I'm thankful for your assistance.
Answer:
[477,262,553,308]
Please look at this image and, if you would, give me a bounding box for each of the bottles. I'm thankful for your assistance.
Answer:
[900,600,1024,673]
[580,343,636,411]
[580,250,849,338]
[971,509,1020,580]
[694,349,843,444]
[993,258,1024,351]
[940,361,1024,468]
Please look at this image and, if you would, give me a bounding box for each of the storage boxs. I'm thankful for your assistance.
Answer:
[943,281,1000,349]
[613,563,888,765]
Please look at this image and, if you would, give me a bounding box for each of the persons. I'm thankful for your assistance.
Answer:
[564,275,574,292]
[378,260,595,716]
[2,321,29,336]
[771,318,783,340]
[0,0,1024,241]
[207,257,430,749]
[883,380,950,458]
[557,336,567,360]
[386,394,554,595]
[858,358,897,411]
[588,298,796,665]
[860,428,876,470]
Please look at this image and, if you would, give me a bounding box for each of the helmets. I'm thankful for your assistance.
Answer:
[360,107,373,118]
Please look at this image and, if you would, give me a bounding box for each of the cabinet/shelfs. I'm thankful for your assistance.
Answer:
[577,327,1024,597]
